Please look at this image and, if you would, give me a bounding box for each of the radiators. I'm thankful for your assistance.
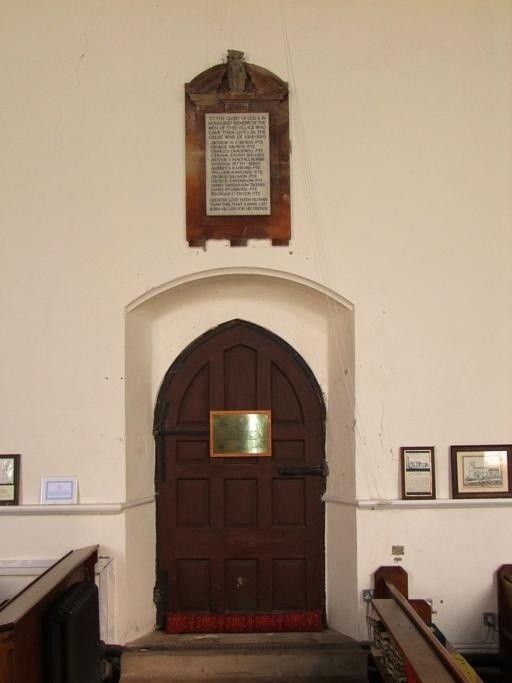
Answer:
[41,580,100,683]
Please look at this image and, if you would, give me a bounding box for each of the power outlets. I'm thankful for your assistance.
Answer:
[483,612,496,626]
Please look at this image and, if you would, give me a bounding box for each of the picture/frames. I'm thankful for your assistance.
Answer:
[449,444,512,499]
[400,445,436,500]
